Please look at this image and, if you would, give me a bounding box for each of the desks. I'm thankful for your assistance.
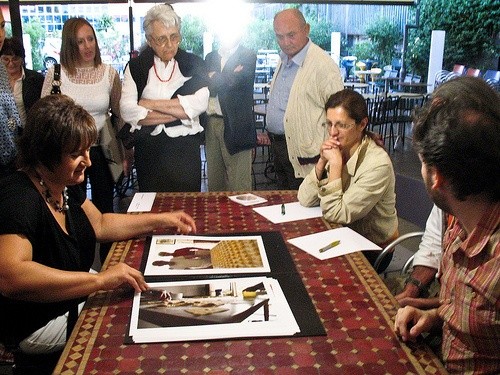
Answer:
[345,82,369,94]
[254,63,276,129]
[354,70,381,93]
[138,295,270,326]
[372,76,403,92]
[51,192,456,375]
[397,82,431,106]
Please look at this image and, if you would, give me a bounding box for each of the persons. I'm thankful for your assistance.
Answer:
[119,4,212,193]
[205,25,257,192]
[158,247,211,259]
[152,255,211,270]
[297,89,399,275]
[264,8,344,191]
[0,37,45,140]
[394,71,461,309]
[0,9,22,179]
[394,75,500,375]
[0,93,197,353]
[41,18,122,266]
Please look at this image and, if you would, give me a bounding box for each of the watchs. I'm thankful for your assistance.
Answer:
[404,277,428,297]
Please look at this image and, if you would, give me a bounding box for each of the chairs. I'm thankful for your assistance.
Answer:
[362,91,475,346]
[252,129,274,187]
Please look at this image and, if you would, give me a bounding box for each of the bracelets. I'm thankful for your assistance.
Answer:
[317,168,323,172]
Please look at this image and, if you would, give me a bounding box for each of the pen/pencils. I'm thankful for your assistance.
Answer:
[319,239,341,253]
[282,203,286,215]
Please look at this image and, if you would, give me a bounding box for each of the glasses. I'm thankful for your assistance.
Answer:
[0,55,23,66]
[321,120,360,130]
[148,33,182,46]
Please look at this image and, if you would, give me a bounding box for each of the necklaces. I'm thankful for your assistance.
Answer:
[154,60,176,82]
[32,167,69,211]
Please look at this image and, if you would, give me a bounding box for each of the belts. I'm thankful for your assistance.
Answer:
[268,133,286,141]
[211,114,223,118]
